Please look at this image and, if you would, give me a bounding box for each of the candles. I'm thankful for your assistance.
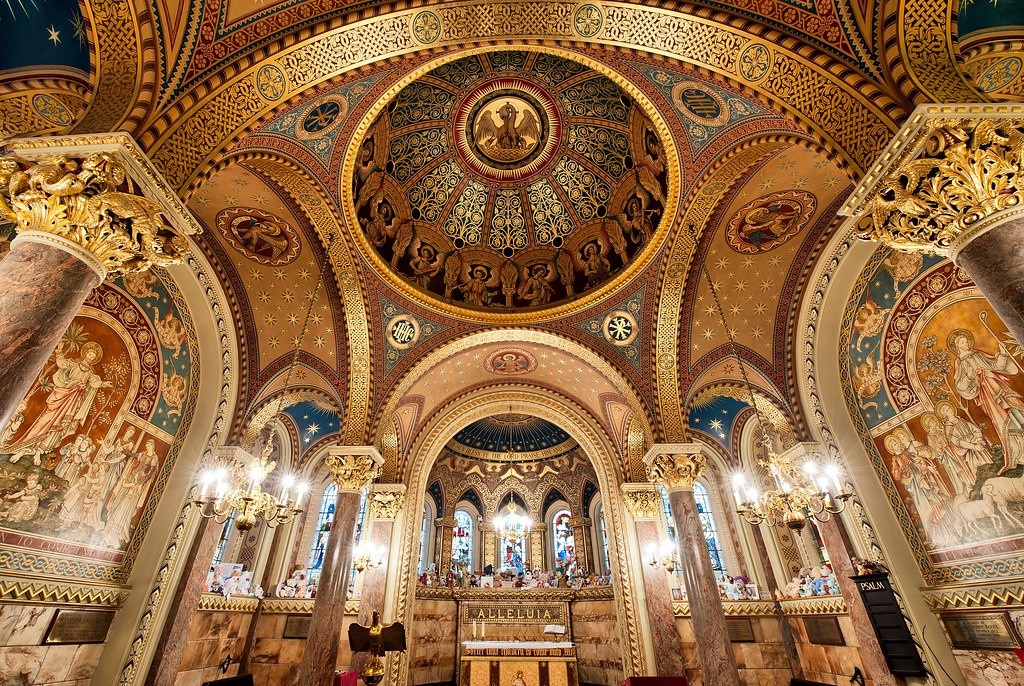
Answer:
[481,622,485,637]
[473,621,477,639]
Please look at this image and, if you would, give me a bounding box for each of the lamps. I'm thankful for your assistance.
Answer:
[689,221,853,535]
[189,231,334,536]
[494,414,533,547]
[643,442,679,574]
